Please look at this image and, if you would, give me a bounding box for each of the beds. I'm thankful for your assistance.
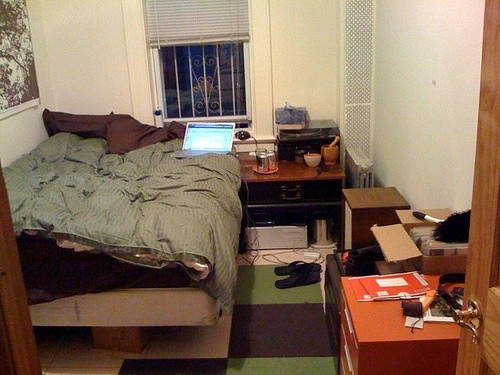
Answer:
[15,134,237,353]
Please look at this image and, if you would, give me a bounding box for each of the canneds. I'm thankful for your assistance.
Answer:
[256,152,269,172]
[266,151,276,171]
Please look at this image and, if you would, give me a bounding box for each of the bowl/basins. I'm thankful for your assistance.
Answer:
[323,145,338,165]
[304,154,321,167]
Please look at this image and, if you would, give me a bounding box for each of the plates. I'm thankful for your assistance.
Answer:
[253,165,278,174]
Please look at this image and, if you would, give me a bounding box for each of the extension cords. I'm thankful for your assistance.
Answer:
[304,252,320,259]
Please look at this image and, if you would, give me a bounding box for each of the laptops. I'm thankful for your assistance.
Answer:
[170,122,235,157]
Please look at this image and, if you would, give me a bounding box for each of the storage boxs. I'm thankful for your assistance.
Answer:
[92,326,156,354]
[245,226,309,250]
[369,207,467,274]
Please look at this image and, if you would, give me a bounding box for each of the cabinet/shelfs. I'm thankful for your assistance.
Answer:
[240,160,346,250]
[340,275,465,375]
[342,187,411,250]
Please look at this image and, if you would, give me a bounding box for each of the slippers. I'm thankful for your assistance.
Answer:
[275,271,320,289]
[274,261,321,276]
